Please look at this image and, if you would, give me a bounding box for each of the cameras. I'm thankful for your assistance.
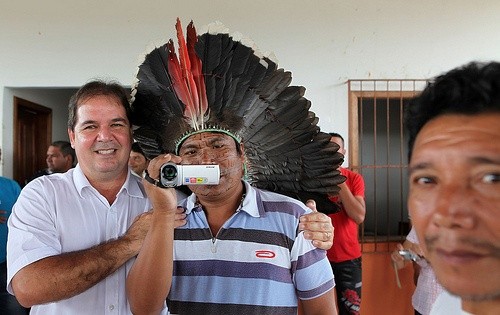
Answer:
[159,162,220,188]
[399,250,421,260]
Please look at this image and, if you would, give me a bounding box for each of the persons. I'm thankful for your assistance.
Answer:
[0,148,21,315]
[124,108,338,314]
[312,132,365,314]
[390,227,440,315]
[404,60,500,315]
[45,141,75,171]
[128,143,148,174]
[7,81,185,315]
